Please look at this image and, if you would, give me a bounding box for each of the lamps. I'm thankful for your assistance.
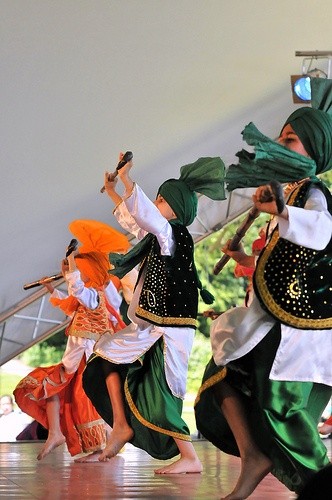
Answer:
[290,55,330,103]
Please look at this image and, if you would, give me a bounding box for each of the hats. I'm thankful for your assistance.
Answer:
[157,178,197,228]
[285,107,330,173]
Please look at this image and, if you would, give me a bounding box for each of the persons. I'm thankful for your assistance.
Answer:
[192,106,332,500]
[0,394,20,442]
[13,246,124,462]
[82,151,204,474]
[203,238,266,320]
